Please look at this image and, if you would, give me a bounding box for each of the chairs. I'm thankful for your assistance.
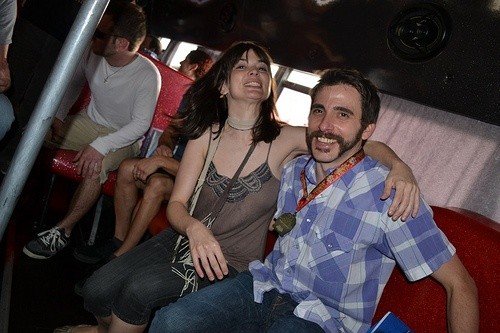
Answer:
[370,204,500,333]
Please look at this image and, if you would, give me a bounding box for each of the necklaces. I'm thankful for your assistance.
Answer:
[103,54,136,84]
[227,122,255,131]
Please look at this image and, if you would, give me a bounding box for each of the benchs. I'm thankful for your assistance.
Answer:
[49,58,195,246]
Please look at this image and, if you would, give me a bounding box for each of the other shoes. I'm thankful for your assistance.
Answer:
[53,324,97,333]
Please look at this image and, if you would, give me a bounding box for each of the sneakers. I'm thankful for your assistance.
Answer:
[75,277,118,296]
[22,224,72,260]
[73,245,111,263]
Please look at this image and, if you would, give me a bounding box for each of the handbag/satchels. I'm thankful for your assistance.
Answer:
[166,232,199,280]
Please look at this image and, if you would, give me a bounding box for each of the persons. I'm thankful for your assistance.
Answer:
[0,0,18,139]
[148,69,479,333]
[142,38,161,56]
[0,0,162,259]
[55,41,420,333]
[179,50,212,80]
[74,91,193,297]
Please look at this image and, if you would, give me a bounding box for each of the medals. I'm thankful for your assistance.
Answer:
[273,212,296,237]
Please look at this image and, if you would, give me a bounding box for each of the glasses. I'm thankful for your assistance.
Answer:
[94,31,129,42]
